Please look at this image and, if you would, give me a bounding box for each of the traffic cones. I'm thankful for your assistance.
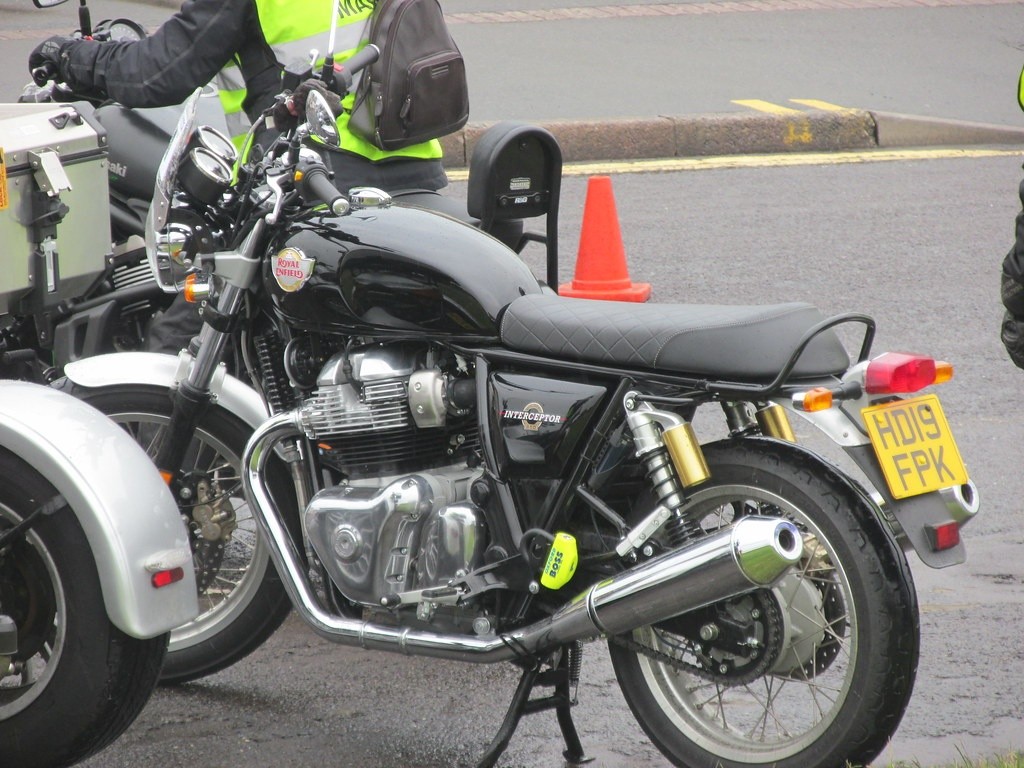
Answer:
[557,177,653,303]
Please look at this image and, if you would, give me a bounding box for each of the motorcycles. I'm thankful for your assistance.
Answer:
[0,378,198,768]
[41,42,980,765]
[0,0,982,767]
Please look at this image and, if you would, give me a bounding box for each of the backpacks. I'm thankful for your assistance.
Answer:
[342,0,469,151]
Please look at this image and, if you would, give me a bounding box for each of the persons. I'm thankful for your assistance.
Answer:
[28,1,449,476]
[1000,65,1024,369]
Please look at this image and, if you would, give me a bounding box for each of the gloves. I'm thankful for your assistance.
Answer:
[28,36,74,87]
